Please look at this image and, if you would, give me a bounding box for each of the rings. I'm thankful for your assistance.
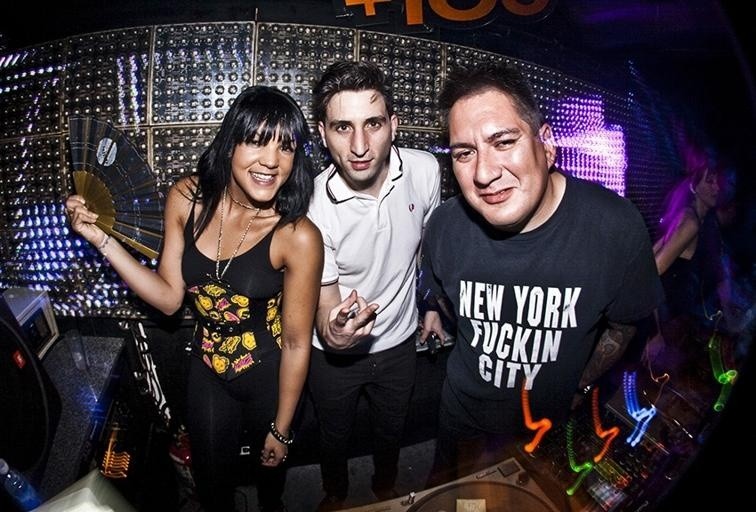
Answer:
[282,458,285,462]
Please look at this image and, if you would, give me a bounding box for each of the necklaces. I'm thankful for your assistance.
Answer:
[215,185,262,288]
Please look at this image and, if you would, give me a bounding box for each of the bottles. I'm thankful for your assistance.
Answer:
[0,459,38,507]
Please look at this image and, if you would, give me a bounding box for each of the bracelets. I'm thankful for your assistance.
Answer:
[98,235,112,252]
[271,420,295,446]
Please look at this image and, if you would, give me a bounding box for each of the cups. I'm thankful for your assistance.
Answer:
[63,329,91,369]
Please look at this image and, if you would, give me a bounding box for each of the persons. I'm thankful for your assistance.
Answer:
[414,62,666,489]
[65,85,323,512]
[640,139,755,368]
[306,61,446,512]
